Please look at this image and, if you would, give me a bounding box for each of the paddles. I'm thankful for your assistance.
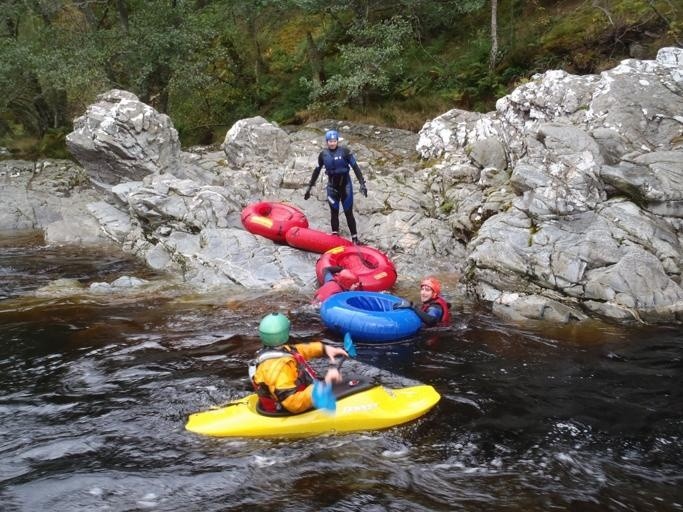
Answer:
[312,332,356,411]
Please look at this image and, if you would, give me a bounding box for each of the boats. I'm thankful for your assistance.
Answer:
[185,377,442,442]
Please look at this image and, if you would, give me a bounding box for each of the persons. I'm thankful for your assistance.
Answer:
[305,130,368,246]
[253,314,350,414]
[394,276,449,326]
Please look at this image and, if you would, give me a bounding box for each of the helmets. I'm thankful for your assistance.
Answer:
[422,278,440,299]
[336,269,359,289]
[258,313,290,346]
[326,130,338,141]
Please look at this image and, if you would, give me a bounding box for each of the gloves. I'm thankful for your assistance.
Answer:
[304,188,312,200]
[393,301,412,311]
[359,181,368,198]
